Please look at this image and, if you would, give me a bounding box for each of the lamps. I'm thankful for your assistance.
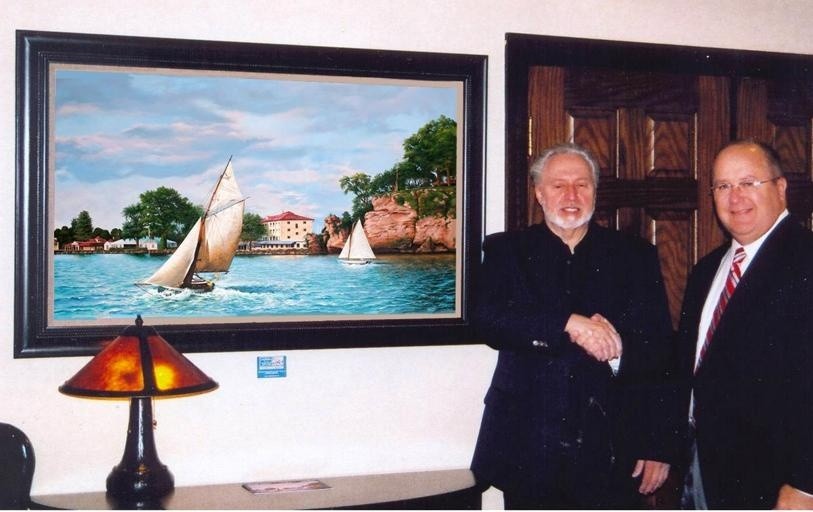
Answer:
[57,315,220,510]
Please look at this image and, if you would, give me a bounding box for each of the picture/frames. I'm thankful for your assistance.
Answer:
[12,28,489,358]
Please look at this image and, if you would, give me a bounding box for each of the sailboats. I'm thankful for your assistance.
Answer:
[338,215,377,266]
[135,154,250,290]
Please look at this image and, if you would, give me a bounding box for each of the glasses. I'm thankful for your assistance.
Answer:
[709,175,782,196]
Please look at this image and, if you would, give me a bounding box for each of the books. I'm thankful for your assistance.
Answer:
[241,478,334,496]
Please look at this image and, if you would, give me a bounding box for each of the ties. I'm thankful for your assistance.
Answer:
[694,248,746,380]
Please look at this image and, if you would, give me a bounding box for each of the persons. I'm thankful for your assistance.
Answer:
[466,142,685,508]
[568,136,812,509]
